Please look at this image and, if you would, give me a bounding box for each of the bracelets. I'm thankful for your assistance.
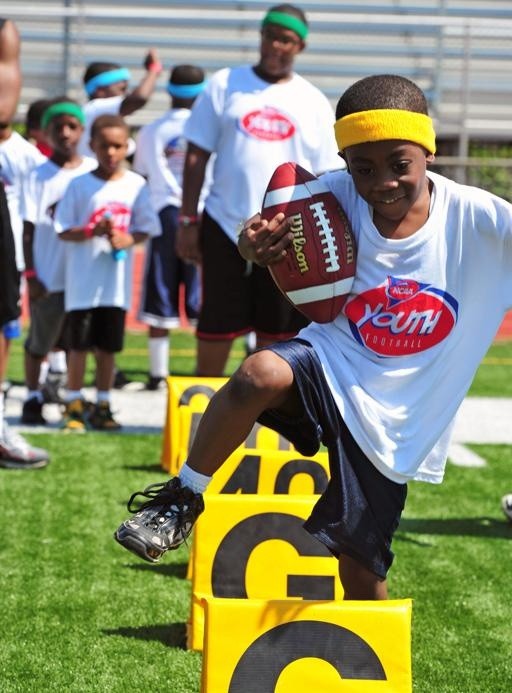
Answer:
[24,270,36,279]
[180,215,196,226]
[85,222,95,238]
[148,64,162,75]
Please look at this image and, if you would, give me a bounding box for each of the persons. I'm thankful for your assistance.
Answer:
[20,97,99,426]
[0,130,50,388]
[114,74,511,600]
[77,46,163,393]
[55,115,164,434]
[0,16,52,469]
[132,63,215,388]
[26,99,69,383]
[181,2,347,375]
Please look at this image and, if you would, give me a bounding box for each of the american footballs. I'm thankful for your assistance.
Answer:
[260,163,356,323]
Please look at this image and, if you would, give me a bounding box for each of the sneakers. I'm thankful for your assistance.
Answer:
[0,421,51,469]
[114,477,205,563]
[59,401,87,434]
[44,379,67,404]
[87,402,123,430]
[21,391,46,424]
